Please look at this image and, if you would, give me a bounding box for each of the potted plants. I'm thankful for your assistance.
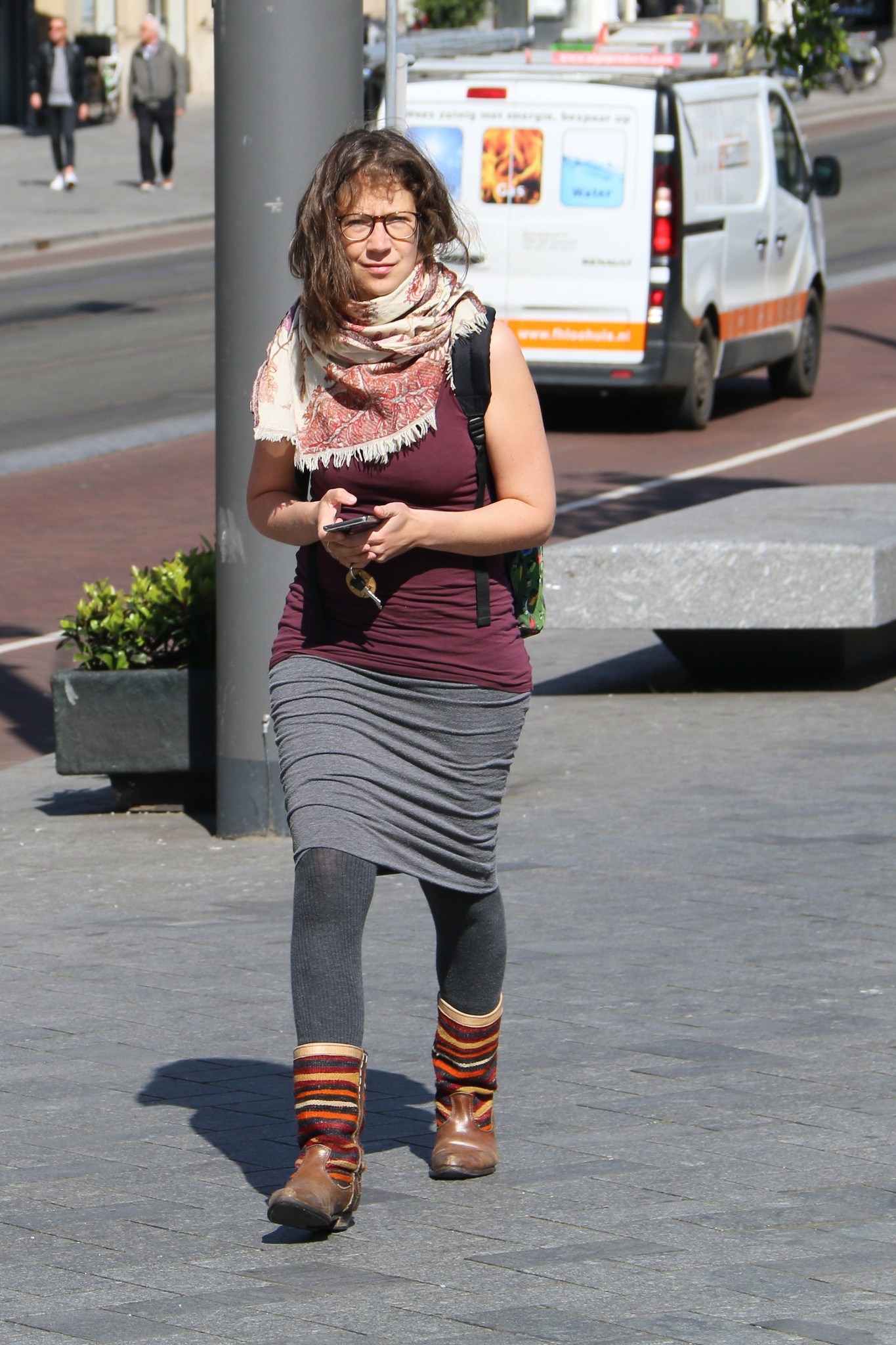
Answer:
[50,535,216,810]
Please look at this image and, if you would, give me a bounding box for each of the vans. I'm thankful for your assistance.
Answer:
[375,40,843,433]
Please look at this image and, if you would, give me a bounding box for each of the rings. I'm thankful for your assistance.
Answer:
[327,541,332,552]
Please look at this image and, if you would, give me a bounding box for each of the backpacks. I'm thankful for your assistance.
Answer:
[450,303,548,639]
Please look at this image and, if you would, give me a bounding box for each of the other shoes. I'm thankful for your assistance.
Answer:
[64,172,77,190]
[50,174,64,191]
[161,177,172,189]
[140,180,152,191]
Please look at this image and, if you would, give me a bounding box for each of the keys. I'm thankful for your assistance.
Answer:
[346,562,382,610]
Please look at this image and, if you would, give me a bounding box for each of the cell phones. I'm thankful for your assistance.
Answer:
[324,516,382,535]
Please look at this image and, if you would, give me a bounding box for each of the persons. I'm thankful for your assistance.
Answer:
[24,16,93,192]
[246,127,558,1234]
[125,13,187,192]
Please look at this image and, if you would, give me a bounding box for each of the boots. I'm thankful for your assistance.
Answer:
[428,989,502,1178]
[266,1042,368,1232]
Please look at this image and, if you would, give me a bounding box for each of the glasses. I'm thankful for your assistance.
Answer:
[333,211,424,243]
